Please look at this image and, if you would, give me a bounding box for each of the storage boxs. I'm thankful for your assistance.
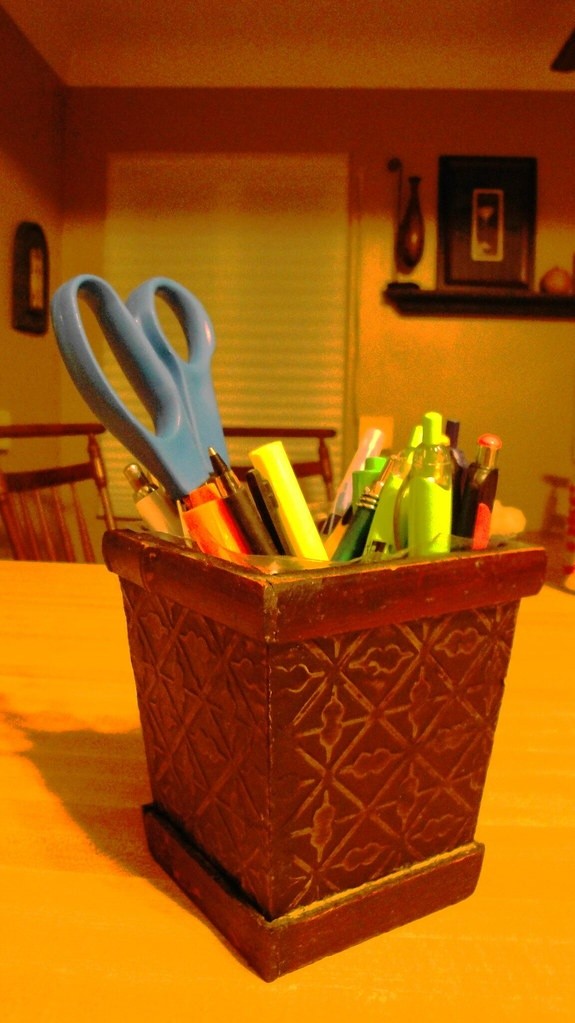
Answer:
[98,513,547,985]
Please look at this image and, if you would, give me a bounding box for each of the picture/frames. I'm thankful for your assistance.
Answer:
[435,149,541,299]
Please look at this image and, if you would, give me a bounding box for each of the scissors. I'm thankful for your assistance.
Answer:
[50,274,229,503]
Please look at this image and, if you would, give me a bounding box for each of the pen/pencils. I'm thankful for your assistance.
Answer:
[123,407,507,570]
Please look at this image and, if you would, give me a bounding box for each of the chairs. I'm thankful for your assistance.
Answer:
[0,421,117,565]
[222,427,337,506]
[516,473,575,594]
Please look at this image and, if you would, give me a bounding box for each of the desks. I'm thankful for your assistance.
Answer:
[1,554,575,1022]
